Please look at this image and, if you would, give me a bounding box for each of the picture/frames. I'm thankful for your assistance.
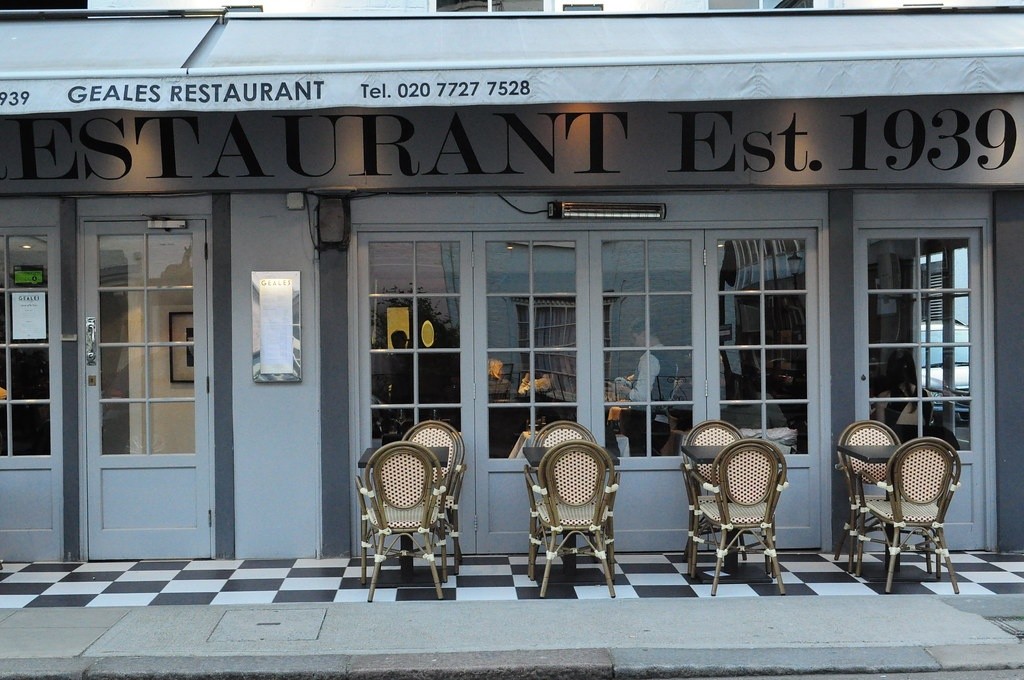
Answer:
[168,311,195,383]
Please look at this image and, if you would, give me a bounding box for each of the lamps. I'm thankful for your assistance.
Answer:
[306,186,357,253]
[547,201,666,221]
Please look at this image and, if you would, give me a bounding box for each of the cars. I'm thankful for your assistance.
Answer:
[918,321,970,422]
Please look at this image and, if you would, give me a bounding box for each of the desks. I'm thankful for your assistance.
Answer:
[523,447,620,588]
[508,431,629,459]
[837,445,937,583]
[680,446,783,584]
[358,447,450,588]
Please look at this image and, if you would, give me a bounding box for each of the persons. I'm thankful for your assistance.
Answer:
[409,322,460,422]
[873,346,935,426]
[382,328,410,430]
[609,314,679,456]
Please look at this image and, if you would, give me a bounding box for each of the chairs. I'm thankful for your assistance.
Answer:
[834,420,961,595]
[680,419,787,596]
[355,419,467,603]
[524,421,621,598]
